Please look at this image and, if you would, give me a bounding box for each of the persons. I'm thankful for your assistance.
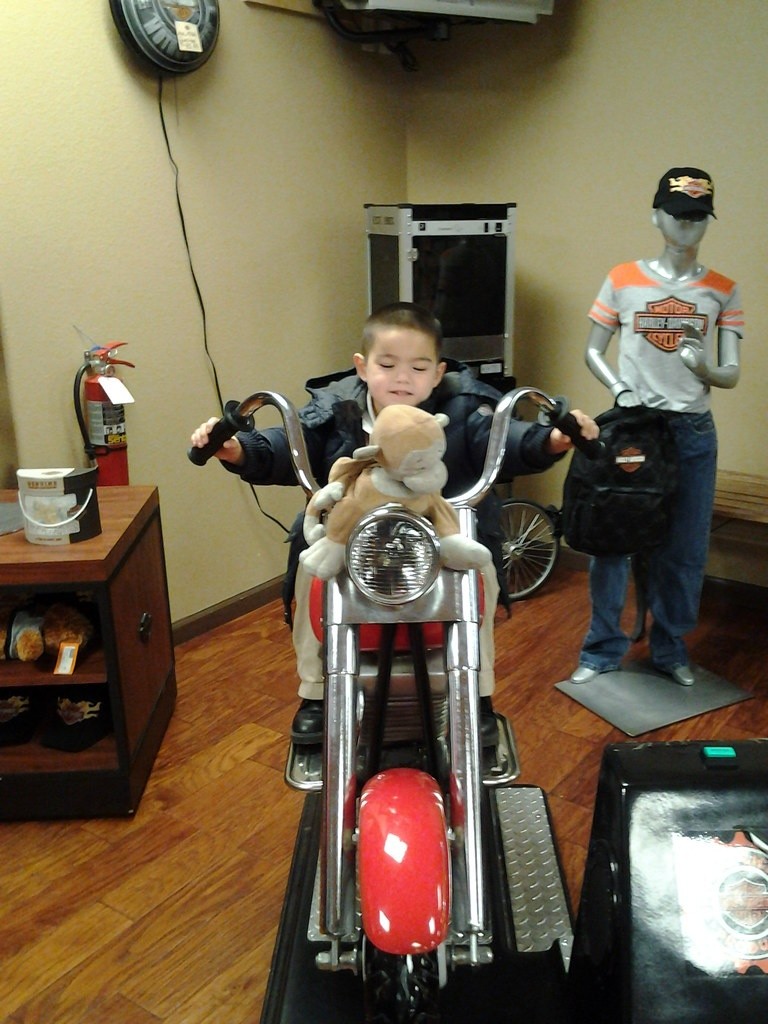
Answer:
[570,166,746,686]
[189,301,599,749]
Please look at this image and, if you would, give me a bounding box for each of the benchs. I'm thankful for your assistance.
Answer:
[632,467,767,644]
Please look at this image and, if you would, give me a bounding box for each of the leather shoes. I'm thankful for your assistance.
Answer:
[290,698,325,745]
[479,696,498,747]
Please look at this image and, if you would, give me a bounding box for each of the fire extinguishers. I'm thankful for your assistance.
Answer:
[73,340,137,487]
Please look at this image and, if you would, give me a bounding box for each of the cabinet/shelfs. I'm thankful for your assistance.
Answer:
[0,483,178,824]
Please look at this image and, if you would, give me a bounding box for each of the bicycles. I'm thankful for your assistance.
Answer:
[493,496,564,600]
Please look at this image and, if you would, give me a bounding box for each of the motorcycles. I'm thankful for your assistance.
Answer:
[195,390,605,1024]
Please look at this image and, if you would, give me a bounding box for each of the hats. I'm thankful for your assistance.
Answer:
[653,167,717,220]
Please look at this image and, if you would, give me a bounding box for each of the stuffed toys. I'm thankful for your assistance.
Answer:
[298,404,491,581]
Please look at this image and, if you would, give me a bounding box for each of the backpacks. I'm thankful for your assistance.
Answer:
[553,381,682,559]
